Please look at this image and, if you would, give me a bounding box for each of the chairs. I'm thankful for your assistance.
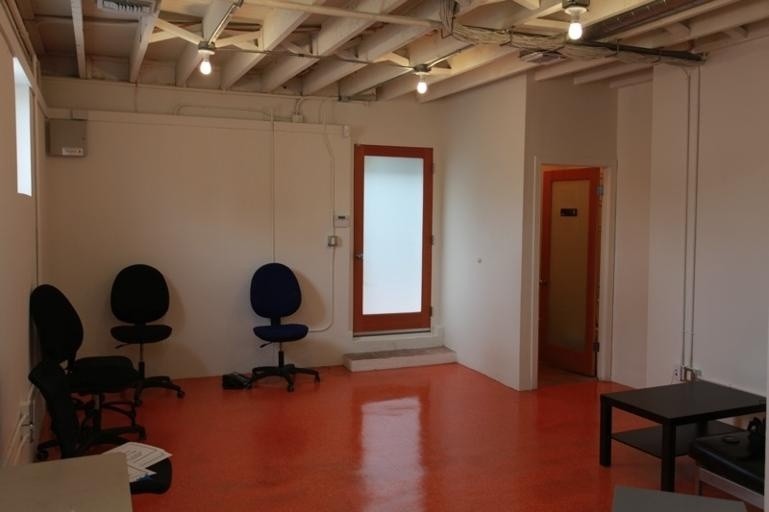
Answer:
[248,263,321,392]
[27,264,186,494]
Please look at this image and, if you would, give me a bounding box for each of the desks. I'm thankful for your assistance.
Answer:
[599,379,765,492]
